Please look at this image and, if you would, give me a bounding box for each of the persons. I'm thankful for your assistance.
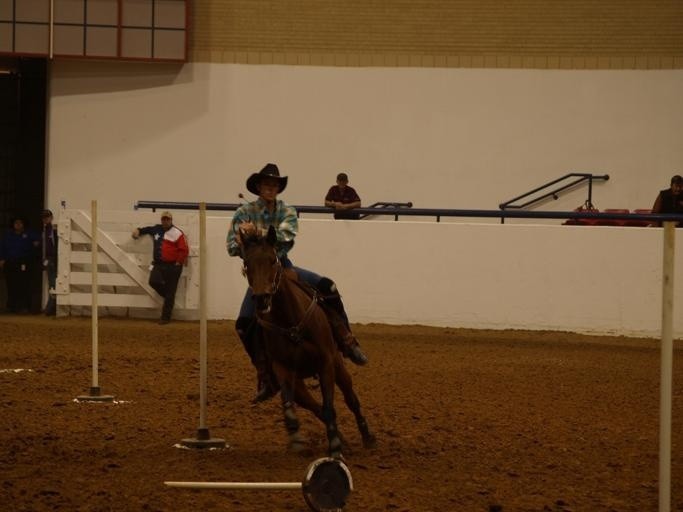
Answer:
[128,210,189,325]
[38,208,57,316]
[654,175,683,226]
[322,172,363,220]
[222,161,369,405]
[0,214,42,316]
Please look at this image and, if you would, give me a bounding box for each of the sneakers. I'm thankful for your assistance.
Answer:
[342,341,368,365]
[250,380,279,403]
[158,318,170,326]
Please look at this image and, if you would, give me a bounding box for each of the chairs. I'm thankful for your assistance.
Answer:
[596,208,630,225]
[563,208,603,225]
[629,209,662,227]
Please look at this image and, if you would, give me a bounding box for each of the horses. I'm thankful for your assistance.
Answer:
[238,224,375,460]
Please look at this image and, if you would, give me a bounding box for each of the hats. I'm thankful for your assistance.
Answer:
[39,209,53,217]
[161,211,172,219]
[246,163,288,195]
